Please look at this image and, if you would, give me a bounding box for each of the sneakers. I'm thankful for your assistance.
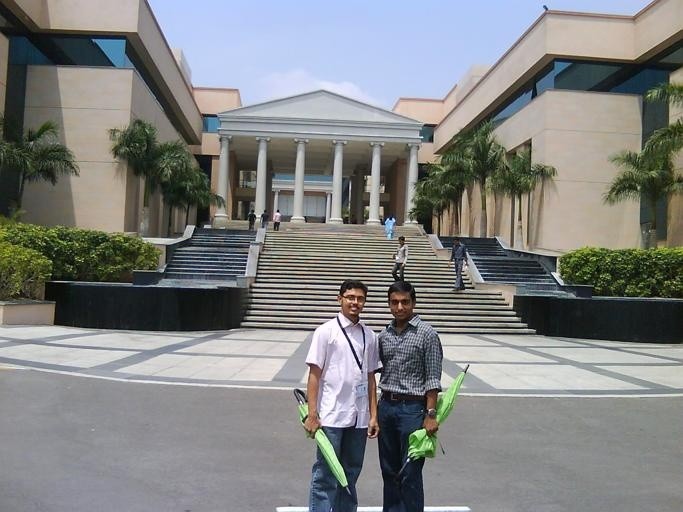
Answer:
[452,287,465,291]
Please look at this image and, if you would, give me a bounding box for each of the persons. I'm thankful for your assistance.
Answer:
[385,214,396,240]
[273,209,281,231]
[247,210,256,232]
[391,236,408,282]
[304,279,384,512]
[448,236,469,291]
[260,210,269,228]
[376,282,443,512]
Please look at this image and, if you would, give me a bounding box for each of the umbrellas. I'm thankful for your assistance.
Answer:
[397,364,469,485]
[294,389,358,505]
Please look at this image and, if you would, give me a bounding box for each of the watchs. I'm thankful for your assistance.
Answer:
[424,408,437,416]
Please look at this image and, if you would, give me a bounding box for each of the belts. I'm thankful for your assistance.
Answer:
[384,391,426,401]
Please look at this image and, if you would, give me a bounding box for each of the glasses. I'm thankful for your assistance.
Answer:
[342,295,364,302]
